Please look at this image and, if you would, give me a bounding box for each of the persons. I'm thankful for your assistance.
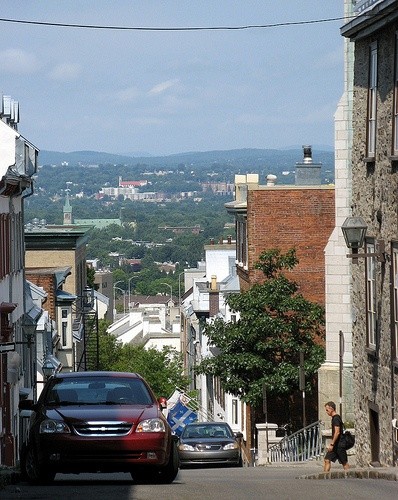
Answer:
[324,402,350,472]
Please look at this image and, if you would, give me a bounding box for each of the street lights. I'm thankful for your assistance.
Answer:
[160,282,172,308]
[113,280,125,321]
[113,286,125,317]
[129,276,139,314]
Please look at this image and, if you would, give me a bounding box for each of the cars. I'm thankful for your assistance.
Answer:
[18,371,180,486]
[174,420,243,468]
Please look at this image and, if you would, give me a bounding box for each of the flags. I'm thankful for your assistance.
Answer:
[162,387,199,437]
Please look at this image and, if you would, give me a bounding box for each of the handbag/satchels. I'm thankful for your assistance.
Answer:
[338,431,355,451]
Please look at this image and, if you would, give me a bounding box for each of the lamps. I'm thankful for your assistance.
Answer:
[341,217,385,264]
[16,317,38,348]
[37,361,55,383]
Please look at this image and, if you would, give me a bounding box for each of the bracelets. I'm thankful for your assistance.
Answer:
[330,444,335,446]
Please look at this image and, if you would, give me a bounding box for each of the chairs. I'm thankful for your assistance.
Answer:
[55,390,78,402]
[113,387,133,403]
